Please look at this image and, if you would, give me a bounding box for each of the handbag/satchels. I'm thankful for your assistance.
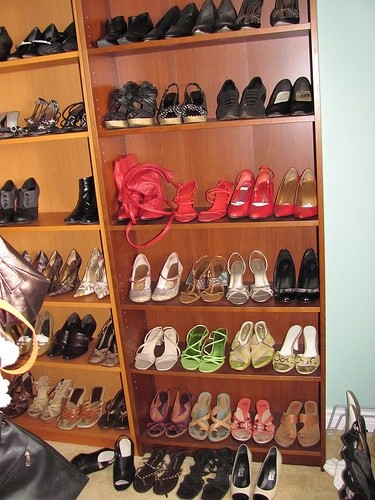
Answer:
[0,231,50,330]
[0,414,90,500]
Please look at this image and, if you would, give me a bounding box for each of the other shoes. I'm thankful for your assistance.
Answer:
[0,0,321,448]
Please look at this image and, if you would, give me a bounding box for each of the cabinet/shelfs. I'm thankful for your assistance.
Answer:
[0,0,327,472]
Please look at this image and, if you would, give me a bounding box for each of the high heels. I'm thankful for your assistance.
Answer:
[112,435,136,491]
[230,443,253,500]
[71,447,116,475]
[253,446,283,500]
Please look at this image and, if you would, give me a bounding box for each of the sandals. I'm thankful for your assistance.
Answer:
[177,448,233,500]
[133,446,191,496]
